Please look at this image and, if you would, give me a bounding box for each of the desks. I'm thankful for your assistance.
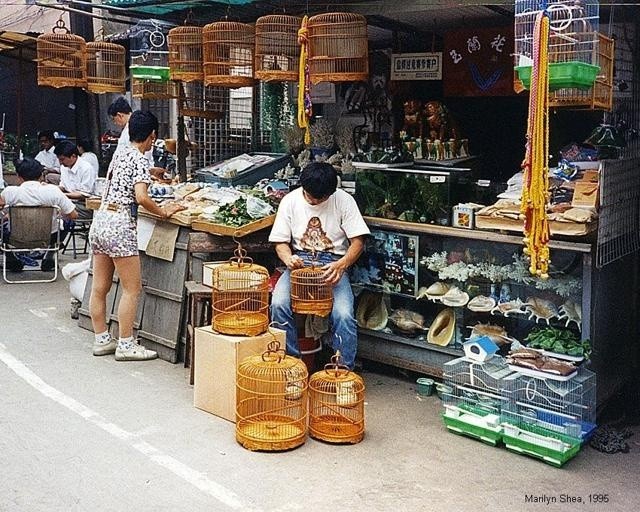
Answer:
[183,280,265,385]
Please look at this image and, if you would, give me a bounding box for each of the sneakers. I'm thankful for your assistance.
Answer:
[335,381,359,410]
[92,339,119,356]
[284,365,306,402]
[2,243,64,271]
[113,343,158,361]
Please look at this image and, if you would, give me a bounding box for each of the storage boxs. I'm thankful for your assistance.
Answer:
[195,327,287,423]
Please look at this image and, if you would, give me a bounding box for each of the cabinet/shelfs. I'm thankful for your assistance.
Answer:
[353,215,591,384]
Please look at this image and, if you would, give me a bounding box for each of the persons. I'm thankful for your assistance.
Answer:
[47,139,96,252]
[107,98,158,168]
[75,140,102,176]
[33,130,61,186]
[268,159,375,409]
[1,157,81,272]
[87,108,190,363]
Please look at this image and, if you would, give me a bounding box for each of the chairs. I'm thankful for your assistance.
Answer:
[1,177,110,283]
